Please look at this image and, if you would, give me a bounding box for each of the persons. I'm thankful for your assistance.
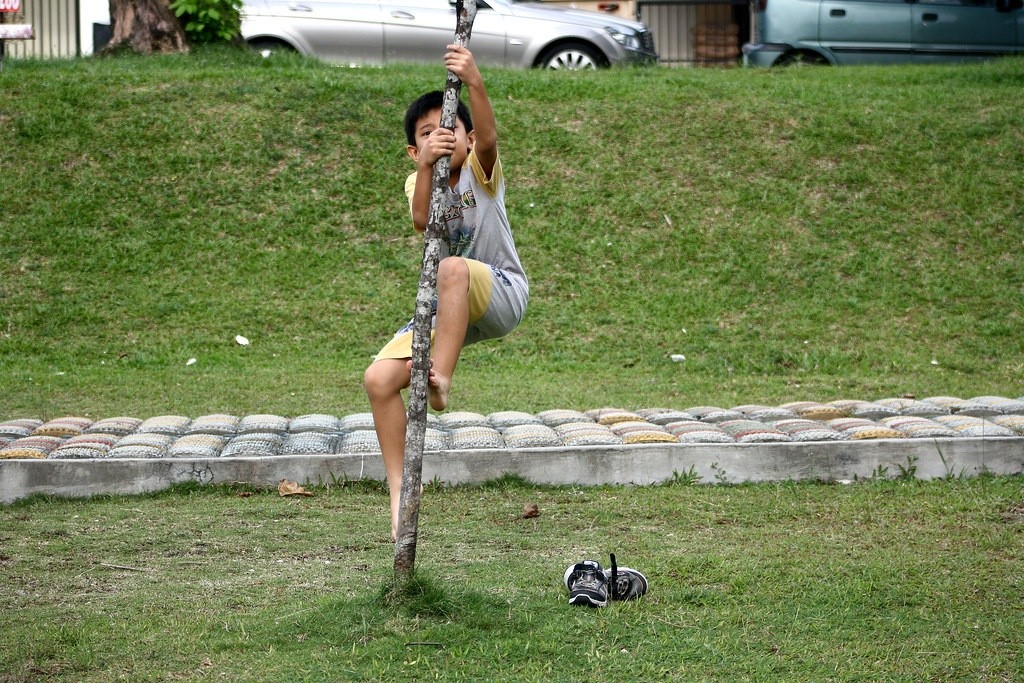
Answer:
[363,44,529,542]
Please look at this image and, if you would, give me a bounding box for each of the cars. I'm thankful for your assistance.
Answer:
[741,1,1024,71]
[231,0,659,73]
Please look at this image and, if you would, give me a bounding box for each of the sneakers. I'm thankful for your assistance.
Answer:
[563,551,648,600]
[563,559,608,606]
